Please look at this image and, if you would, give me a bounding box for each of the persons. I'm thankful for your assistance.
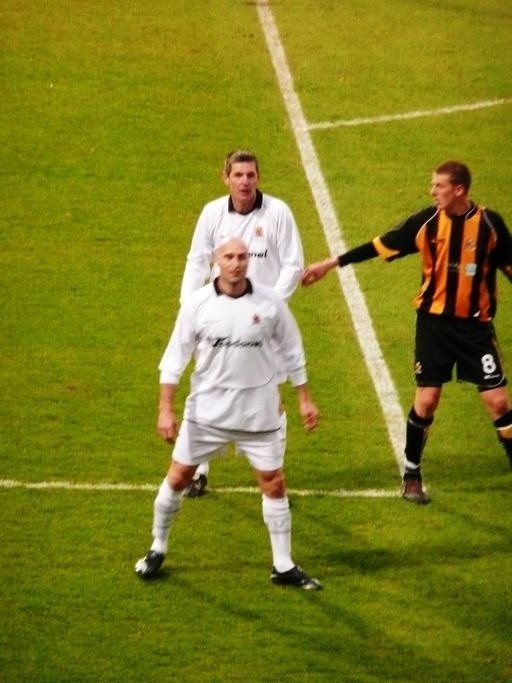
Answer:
[297,159,512,506]
[175,143,308,500]
[130,235,323,592]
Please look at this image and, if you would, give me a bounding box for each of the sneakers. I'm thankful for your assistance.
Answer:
[135,550,165,580]
[182,474,207,497]
[403,472,431,504]
[270,565,320,591]
